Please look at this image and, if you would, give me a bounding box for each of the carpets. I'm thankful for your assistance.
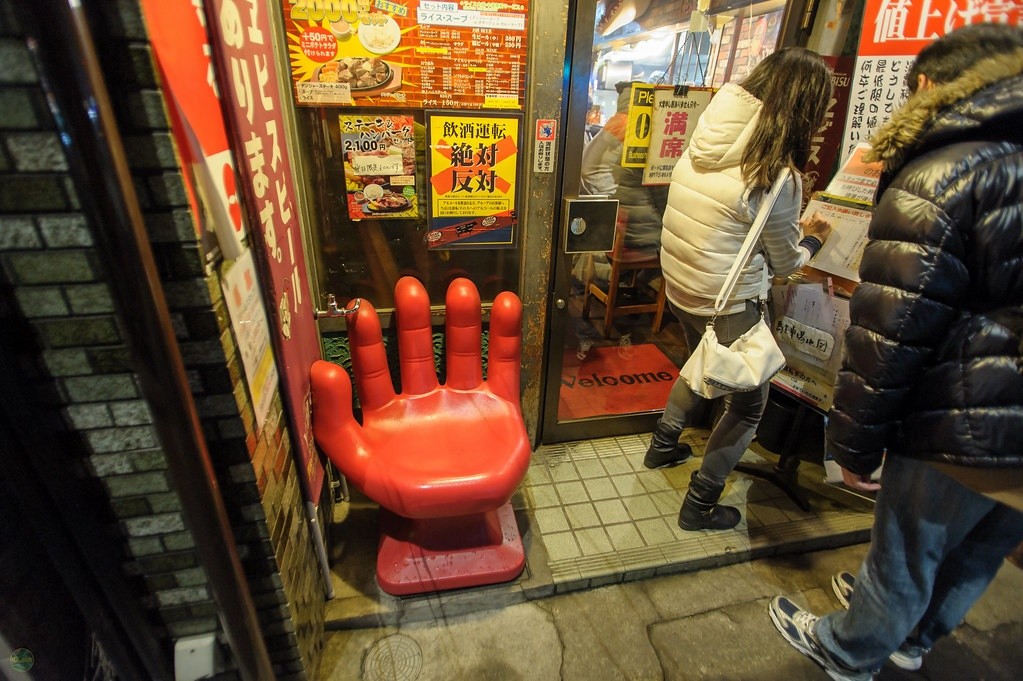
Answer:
[558,345,680,420]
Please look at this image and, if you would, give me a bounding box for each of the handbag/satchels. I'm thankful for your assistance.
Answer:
[678,314,788,399]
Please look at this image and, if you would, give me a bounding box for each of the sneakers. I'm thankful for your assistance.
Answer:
[831,569,924,670]
[768,594,874,681]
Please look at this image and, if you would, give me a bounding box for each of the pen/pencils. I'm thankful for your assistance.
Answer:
[793,217,807,225]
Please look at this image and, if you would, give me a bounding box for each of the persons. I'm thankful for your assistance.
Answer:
[578,71,670,333]
[644,46,832,530]
[768,21,1022,681]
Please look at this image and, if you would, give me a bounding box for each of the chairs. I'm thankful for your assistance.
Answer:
[309,276,531,595]
[582,209,665,339]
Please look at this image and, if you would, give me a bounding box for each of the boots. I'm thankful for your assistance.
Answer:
[643,418,692,470]
[678,470,741,531]
